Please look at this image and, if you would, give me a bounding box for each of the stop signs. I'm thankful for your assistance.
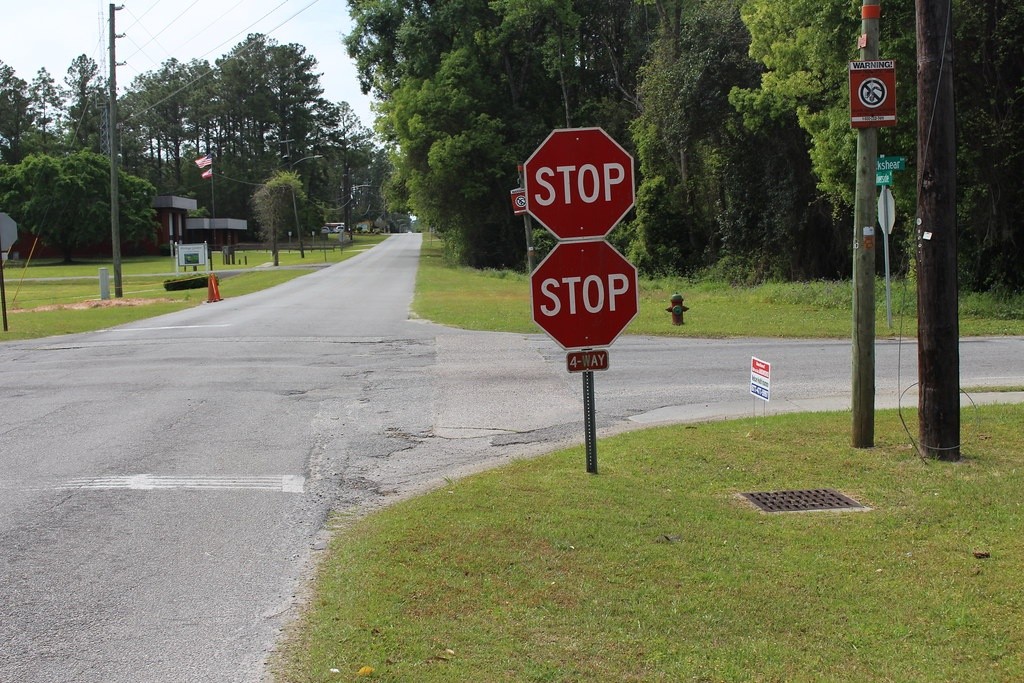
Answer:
[530,240,638,351]
[524,126,636,241]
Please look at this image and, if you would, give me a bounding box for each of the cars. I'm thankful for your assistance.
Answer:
[321,226,379,234]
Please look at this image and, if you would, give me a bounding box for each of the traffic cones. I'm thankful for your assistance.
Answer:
[211,272,223,301]
[207,273,217,303]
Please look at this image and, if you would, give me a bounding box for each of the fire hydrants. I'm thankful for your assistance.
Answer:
[665,291,689,326]
